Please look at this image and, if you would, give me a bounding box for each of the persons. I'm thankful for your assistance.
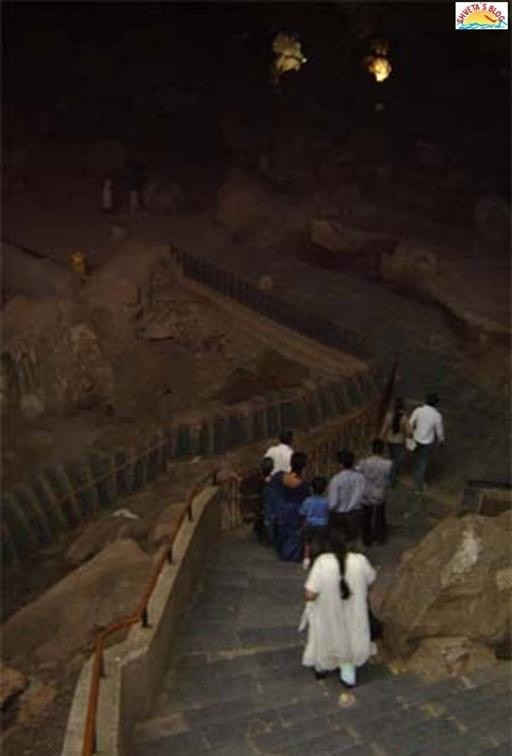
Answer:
[297,525,379,689]
[254,393,447,572]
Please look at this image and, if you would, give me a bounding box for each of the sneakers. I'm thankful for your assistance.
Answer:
[311,666,329,678]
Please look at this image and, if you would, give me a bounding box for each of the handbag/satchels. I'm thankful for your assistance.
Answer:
[404,438,418,452]
